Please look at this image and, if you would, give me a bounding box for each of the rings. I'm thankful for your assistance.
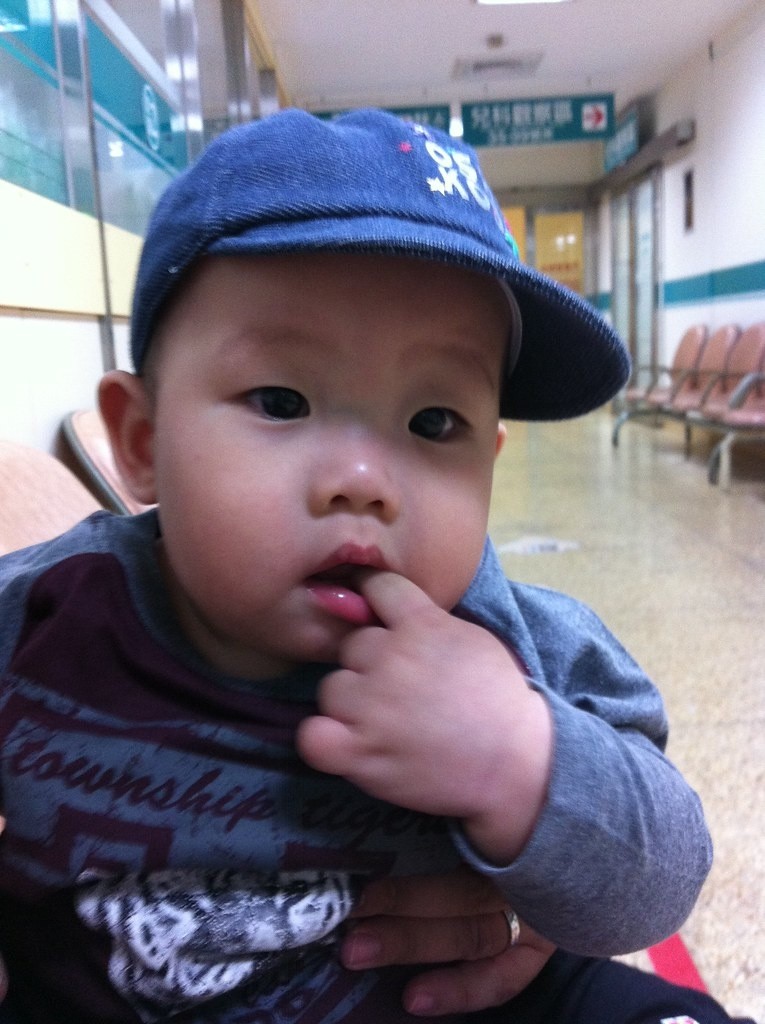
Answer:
[502,908,521,946]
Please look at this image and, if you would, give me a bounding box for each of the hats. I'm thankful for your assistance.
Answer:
[130,108,629,423]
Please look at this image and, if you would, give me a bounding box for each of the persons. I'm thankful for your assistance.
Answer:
[0,106,756,1024]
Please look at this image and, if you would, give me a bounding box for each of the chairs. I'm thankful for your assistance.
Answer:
[61,408,160,515]
[0,439,103,556]
[609,321,765,492]
